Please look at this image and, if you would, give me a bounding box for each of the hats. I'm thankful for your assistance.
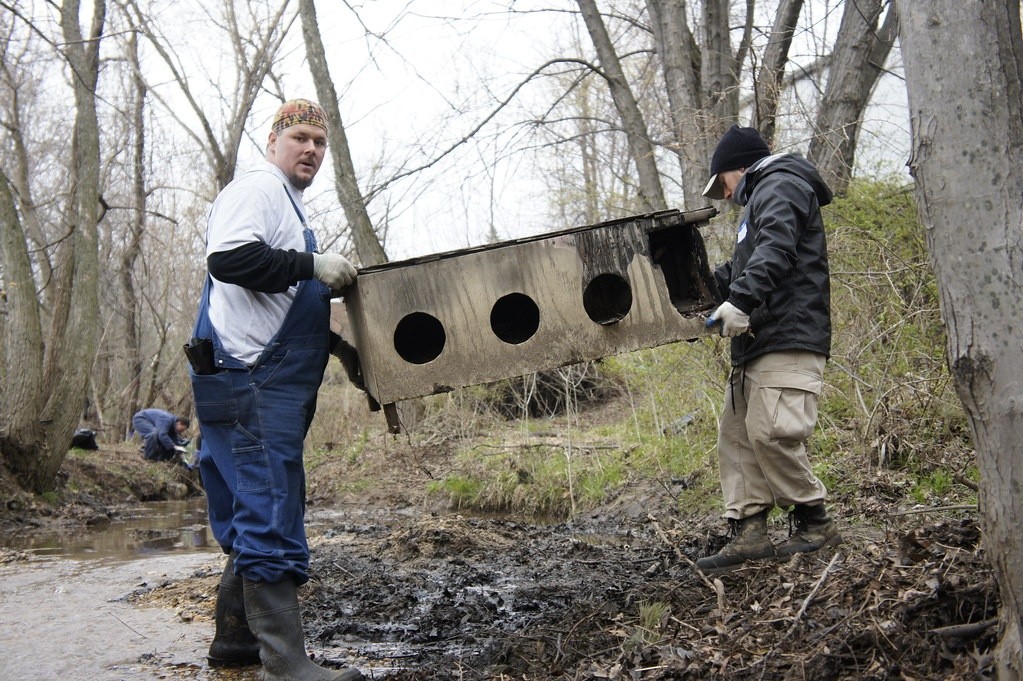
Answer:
[709,124,770,179]
[272,98,328,138]
[702,173,724,199]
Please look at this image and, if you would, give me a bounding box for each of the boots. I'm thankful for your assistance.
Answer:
[205,550,262,667]
[242,575,361,681]
[775,503,842,563]
[187,449,201,471]
[694,508,776,576]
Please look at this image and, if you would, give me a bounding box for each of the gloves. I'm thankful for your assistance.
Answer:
[174,445,188,455]
[330,337,369,394]
[311,252,358,293]
[706,301,750,338]
[181,457,189,469]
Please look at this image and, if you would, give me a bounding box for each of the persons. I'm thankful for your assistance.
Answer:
[183,97,362,681]
[132,408,205,473]
[696,121,844,575]
